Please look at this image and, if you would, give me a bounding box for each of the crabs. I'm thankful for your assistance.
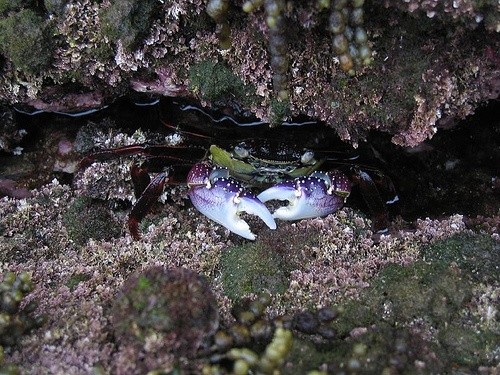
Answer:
[78,92,408,241]
